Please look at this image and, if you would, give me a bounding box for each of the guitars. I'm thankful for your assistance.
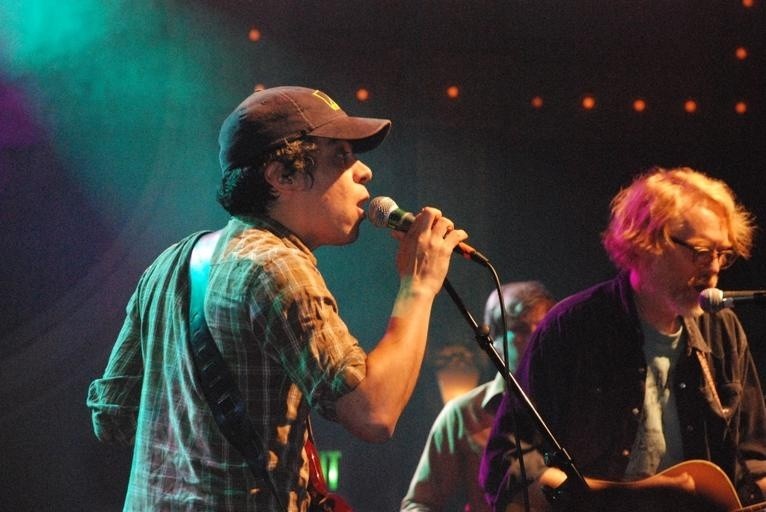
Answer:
[303,435,352,512]
[510,458,766,511]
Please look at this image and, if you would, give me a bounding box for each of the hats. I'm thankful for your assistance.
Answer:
[218,85,393,175]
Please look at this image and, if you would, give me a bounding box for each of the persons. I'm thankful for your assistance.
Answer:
[399,279,555,512]
[478,168,764,512]
[88,87,469,511]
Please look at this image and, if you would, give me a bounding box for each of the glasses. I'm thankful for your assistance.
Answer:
[670,236,738,272]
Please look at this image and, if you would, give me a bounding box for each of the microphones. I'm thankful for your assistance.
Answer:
[699,287,766,312]
[367,196,477,259]
